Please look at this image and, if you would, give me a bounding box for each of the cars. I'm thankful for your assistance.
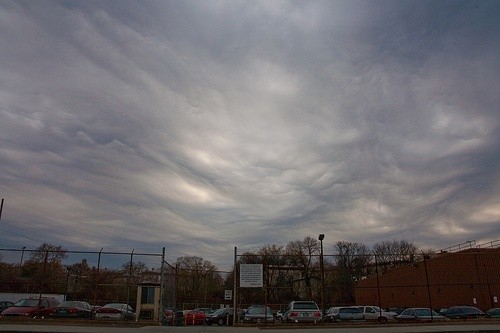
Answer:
[92,306,103,312]
[96,303,136,322]
[384,306,500,321]
[0,296,60,319]
[164,307,220,325]
[52,301,95,320]
[0,301,15,312]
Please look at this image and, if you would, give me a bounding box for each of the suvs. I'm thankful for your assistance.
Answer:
[323,307,366,322]
[243,305,275,323]
[282,300,322,323]
[353,306,398,324]
[277,309,285,322]
[204,307,246,326]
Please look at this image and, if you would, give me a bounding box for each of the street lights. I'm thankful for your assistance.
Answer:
[318,234,324,315]
